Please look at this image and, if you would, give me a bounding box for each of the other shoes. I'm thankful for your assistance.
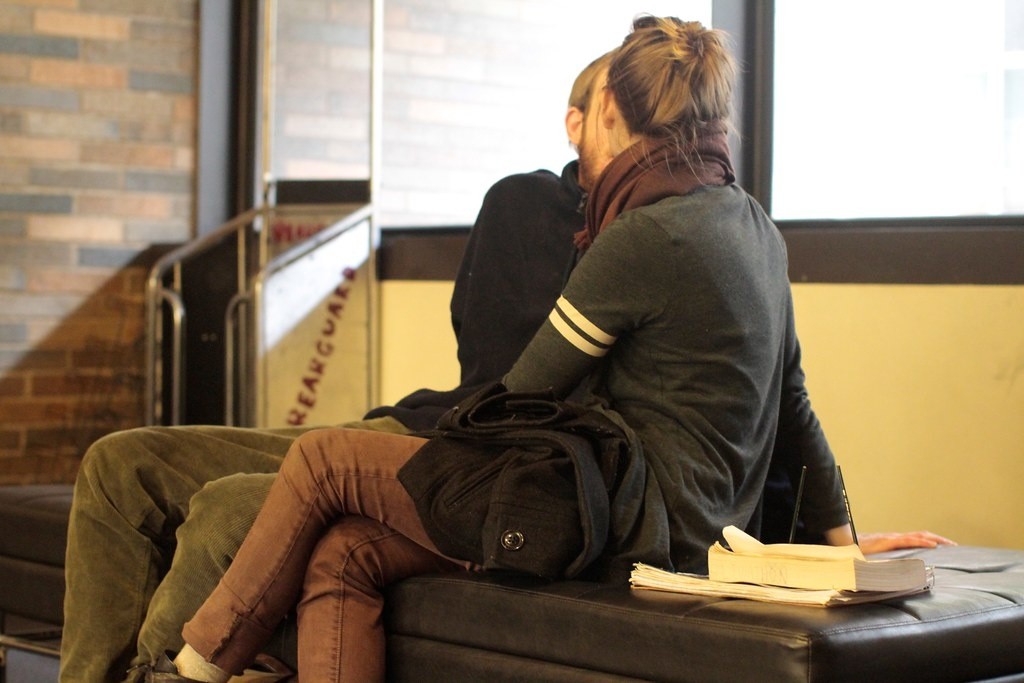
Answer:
[137,651,207,683]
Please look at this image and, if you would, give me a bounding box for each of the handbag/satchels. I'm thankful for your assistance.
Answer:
[397,380,647,576]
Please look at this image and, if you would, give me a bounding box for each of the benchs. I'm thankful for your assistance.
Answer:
[1,484,1024,683]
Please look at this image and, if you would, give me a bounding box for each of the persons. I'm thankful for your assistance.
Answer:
[57,15,958,683]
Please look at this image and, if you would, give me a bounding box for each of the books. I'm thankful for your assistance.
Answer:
[708,525,927,592]
[629,562,935,608]
